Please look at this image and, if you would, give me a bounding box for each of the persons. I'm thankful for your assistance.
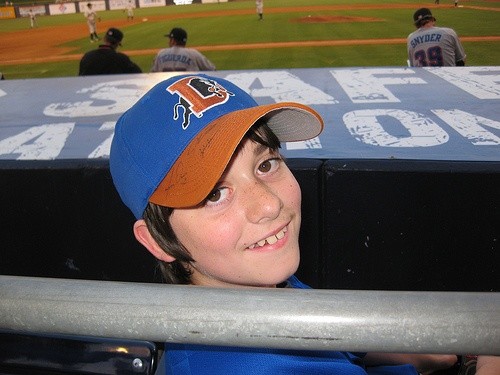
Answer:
[84,2,101,43]
[150,27,219,72]
[28,8,35,27]
[454,0,458,8]
[122,0,137,22]
[256,0,263,21]
[109,73,499,375]
[434,0,440,8]
[78,27,143,77]
[407,7,467,68]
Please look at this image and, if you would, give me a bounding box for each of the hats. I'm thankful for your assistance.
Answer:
[413,8,433,24]
[109,74,324,220]
[164,28,187,43]
[105,28,122,44]
